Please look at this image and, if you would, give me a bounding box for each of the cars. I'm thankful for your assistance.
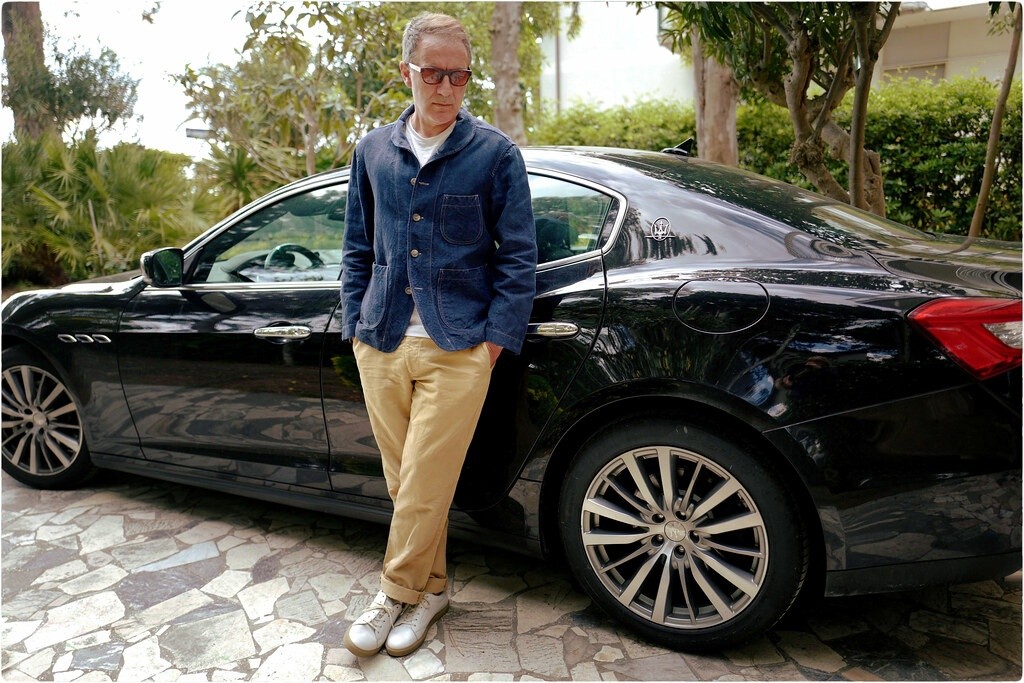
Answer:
[1,142,1023,654]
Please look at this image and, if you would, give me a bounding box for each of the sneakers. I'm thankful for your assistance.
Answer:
[385,591,450,656]
[343,589,403,657]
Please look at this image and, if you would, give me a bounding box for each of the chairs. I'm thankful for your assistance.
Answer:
[534,215,574,265]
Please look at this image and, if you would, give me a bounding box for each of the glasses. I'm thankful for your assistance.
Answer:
[407,63,472,86]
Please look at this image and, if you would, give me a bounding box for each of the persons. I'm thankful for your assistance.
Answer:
[338,10,539,658]
